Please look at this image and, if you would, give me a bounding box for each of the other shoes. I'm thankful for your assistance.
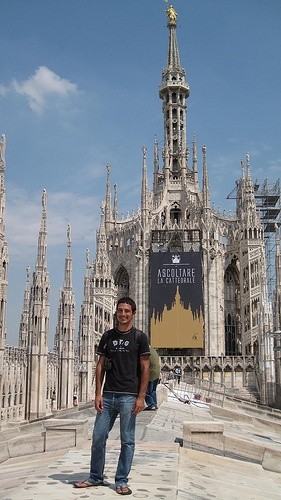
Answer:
[144,405,158,411]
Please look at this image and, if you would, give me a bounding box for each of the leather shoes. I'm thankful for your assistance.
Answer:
[74,479,103,488]
[116,486,132,495]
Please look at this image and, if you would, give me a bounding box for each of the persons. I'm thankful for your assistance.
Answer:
[144,341,160,411]
[167,361,183,384]
[72,297,151,495]
